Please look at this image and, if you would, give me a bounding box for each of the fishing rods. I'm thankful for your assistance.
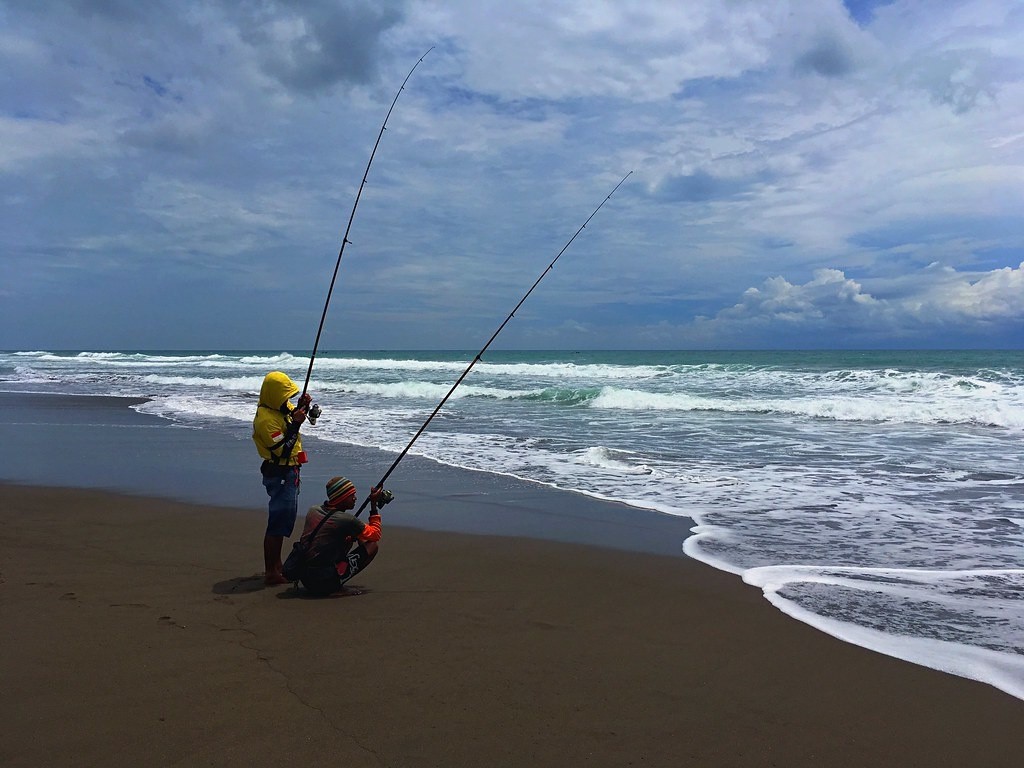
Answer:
[281,40,438,485]
[353,169,635,516]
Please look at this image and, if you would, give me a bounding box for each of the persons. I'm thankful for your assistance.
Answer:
[298,476,384,597]
[250,369,309,584]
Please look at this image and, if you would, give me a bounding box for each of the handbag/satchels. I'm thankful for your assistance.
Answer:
[281,542,308,582]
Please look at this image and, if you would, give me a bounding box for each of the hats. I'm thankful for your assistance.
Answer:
[326,476,357,505]
